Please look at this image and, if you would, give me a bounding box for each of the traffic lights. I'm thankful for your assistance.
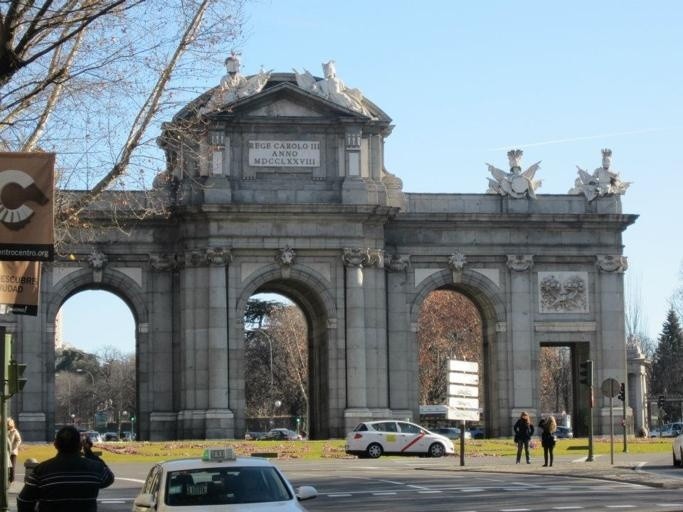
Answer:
[129,416,135,422]
[578,360,593,387]
[7,359,30,396]
[657,396,665,409]
[617,382,625,401]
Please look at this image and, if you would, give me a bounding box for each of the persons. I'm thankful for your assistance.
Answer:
[195,56,247,120]
[538,416,557,468]
[311,61,380,122]
[7,417,22,484]
[15,425,115,512]
[232,468,274,502]
[498,165,536,201]
[583,157,621,195]
[513,412,534,466]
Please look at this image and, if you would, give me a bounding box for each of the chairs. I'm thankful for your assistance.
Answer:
[174,470,269,501]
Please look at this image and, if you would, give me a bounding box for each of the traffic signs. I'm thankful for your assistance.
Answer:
[445,358,481,423]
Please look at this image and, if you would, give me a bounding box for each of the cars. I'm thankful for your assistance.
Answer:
[553,426,574,439]
[246,427,299,442]
[128,446,318,512]
[81,428,135,441]
[647,421,683,468]
[342,420,488,459]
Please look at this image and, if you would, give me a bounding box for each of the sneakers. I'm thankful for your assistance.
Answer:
[542,464,548,467]
[516,461,520,465]
[550,464,553,466]
[527,460,531,464]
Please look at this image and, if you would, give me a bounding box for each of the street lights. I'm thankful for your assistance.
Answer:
[279,397,286,426]
[53,372,72,425]
[75,368,96,427]
[269,400,282,430]
[295,417,300,435]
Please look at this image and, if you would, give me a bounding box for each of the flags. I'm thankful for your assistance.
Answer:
[0,151,54,264]
[0,259,42,317]
